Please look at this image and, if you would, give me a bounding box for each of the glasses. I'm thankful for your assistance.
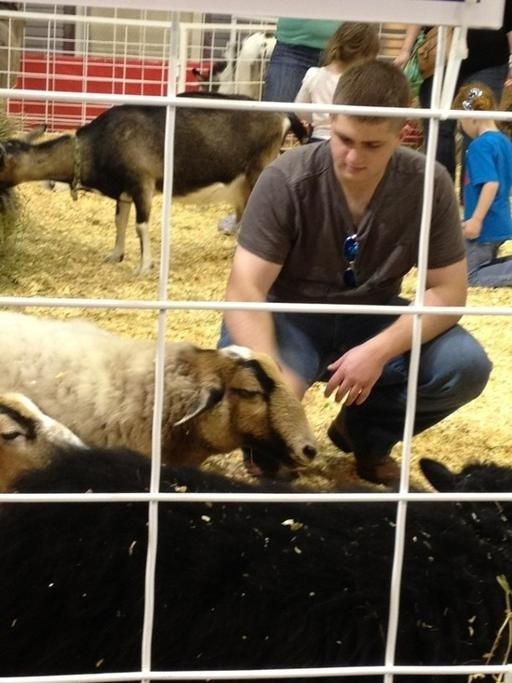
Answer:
[344,236,359,290]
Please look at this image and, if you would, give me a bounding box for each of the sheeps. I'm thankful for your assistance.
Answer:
[0,308,320,510]
[191,32,277,100]
[0,444,512,683]
[0,90,292,278]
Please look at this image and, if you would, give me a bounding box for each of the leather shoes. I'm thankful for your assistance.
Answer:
[329,409,399,482]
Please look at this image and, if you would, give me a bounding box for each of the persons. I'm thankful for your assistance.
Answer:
[218,16,344,238]
[391,0,511,224]
[452,82,512,289]
[293,21,379,144]
[216,60,493,485]
